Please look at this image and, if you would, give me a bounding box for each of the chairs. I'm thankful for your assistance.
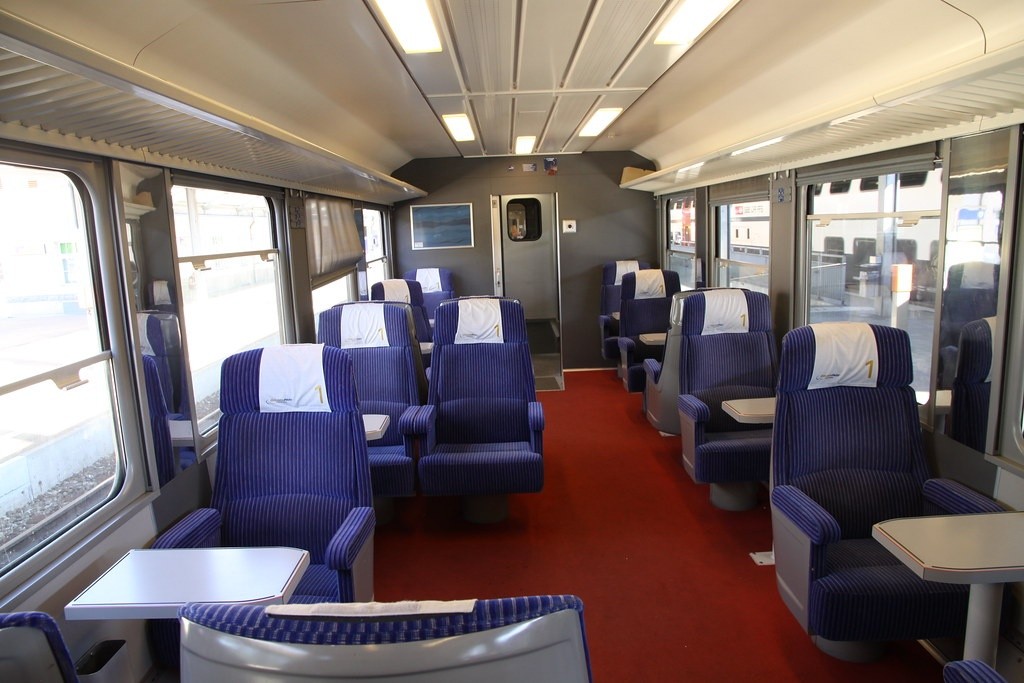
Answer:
[949,316,1000,455]
[769,323,1008,661]
[0,611,82,683]
[316,259,543,522]
[938,262,1000,390]
[943,659,1008,683]
[154,340,375,603]
[179,595,594,683]
[600,259,781,512]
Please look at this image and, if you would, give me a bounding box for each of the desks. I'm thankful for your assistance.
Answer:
[612,311,620,321]
[639,333,667,345]
[360,318,434,442]
[722,396,776,424]
[914,389,952,436]
[871,512,1023,672]
[64,546,310,620]
